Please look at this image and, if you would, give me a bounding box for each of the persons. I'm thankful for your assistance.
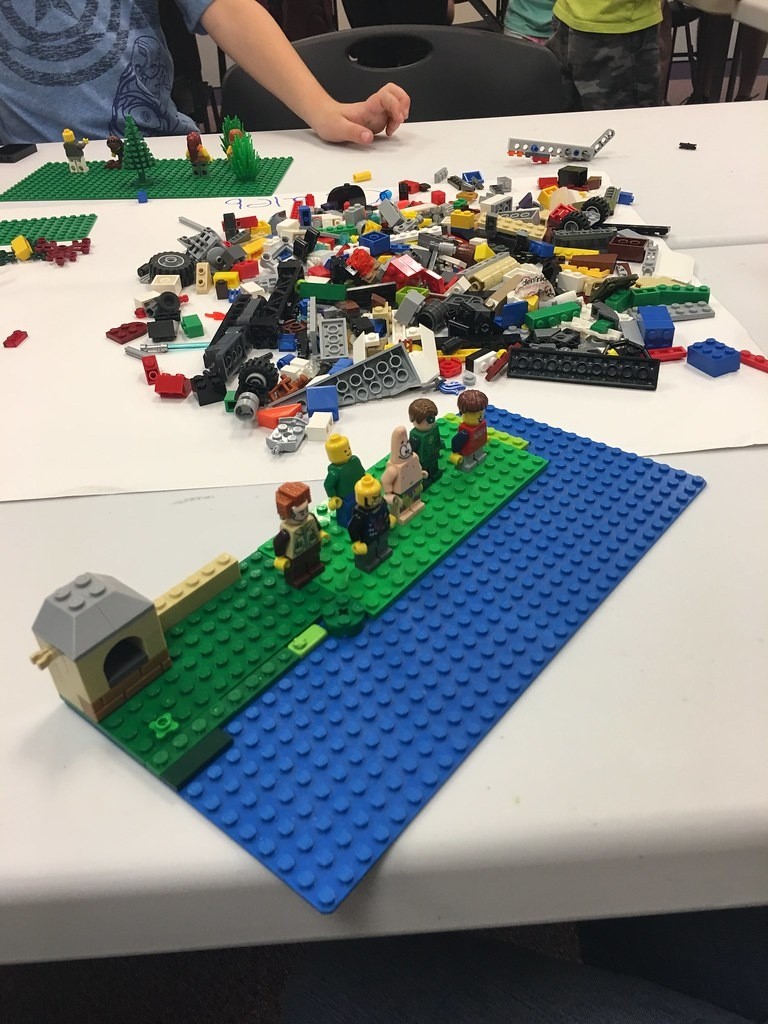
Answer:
[451,389,489,472]
[225,129,243,162]
[0,0,454,145]
[323,398,444,572]
[274,482,328,590]
[62,128,89,172]
[503,0,768,112]
[185,131,213,177]
[105,136,126,170]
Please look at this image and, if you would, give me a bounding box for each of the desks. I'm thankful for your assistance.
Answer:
[1,103,768,961]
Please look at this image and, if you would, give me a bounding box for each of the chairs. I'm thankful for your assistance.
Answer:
[221,24,570,132]
